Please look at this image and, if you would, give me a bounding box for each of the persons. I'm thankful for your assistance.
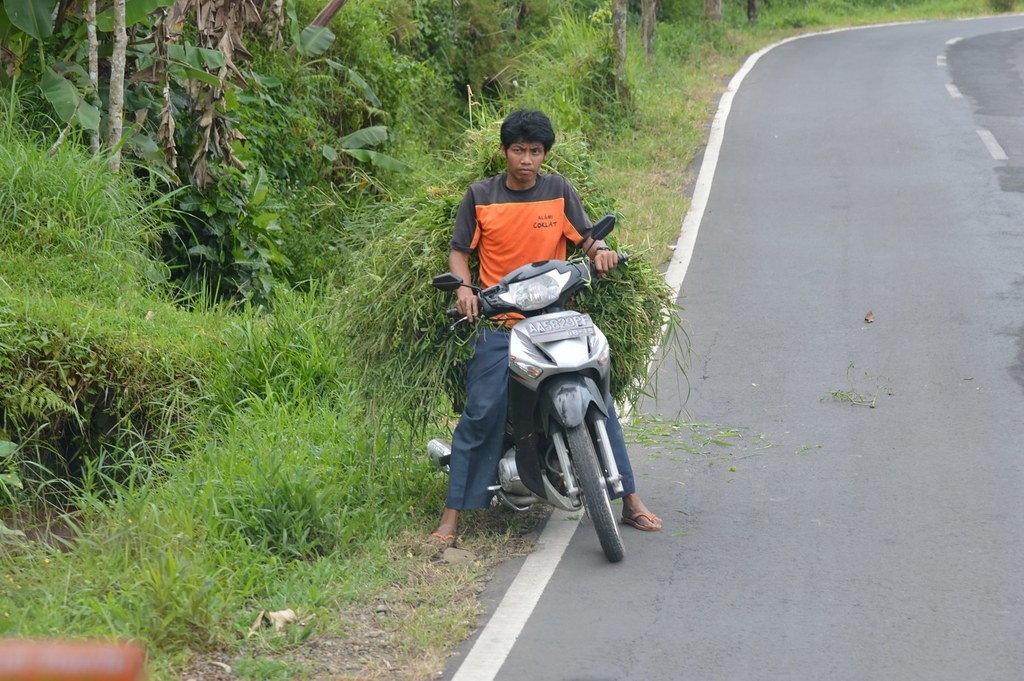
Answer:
[425,109,663,548]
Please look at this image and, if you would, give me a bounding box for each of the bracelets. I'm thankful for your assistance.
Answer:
[596,245,612,251]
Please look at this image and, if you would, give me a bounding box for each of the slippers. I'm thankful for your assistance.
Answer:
[426,533,456,558]
[620,512,662,531]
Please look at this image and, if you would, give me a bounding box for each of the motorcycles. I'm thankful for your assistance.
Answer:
[422,219,629,563]
